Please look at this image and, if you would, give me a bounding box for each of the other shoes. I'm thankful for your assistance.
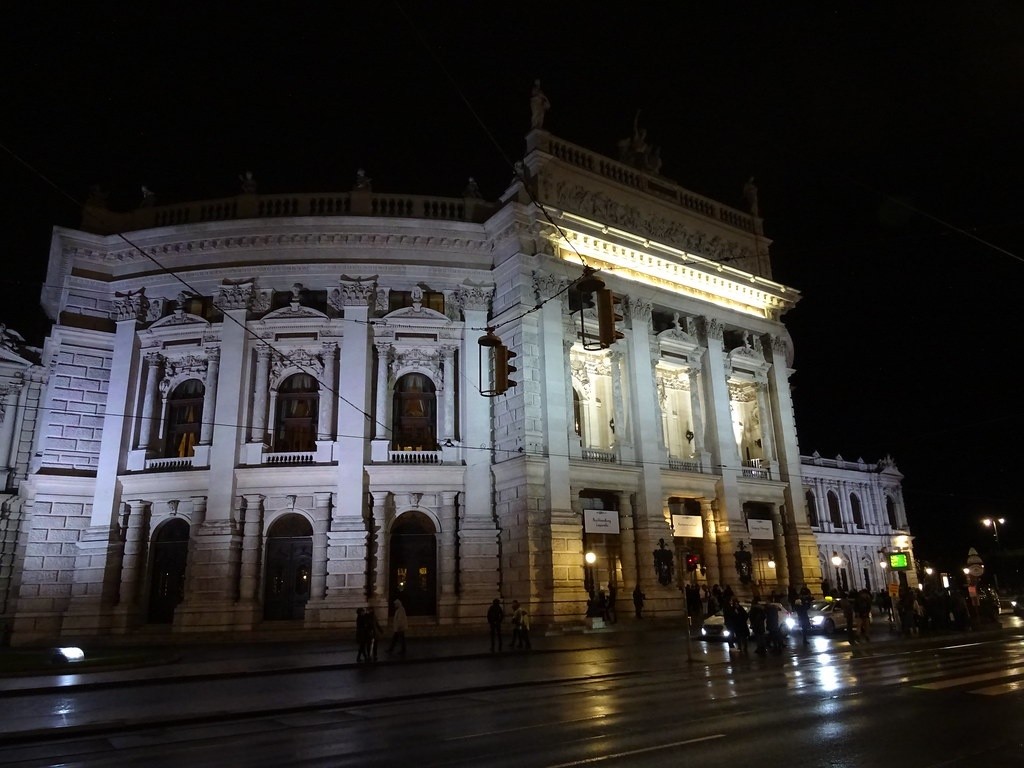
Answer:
[498,648,502,651]
[489,648,494,652]
[397,652,406,656]
[729,642,734,648]
[385,650,392,656]
[509,644,514,647]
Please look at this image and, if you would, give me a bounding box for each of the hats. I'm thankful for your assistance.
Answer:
[730,599,740,604]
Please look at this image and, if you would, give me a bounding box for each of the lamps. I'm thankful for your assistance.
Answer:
[48,645,85,663]
[686,429,694,444]
[610,417,615,433]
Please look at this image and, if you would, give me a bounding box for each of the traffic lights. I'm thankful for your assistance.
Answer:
[685,553,697,572]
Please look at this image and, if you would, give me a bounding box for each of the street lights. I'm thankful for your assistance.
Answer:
[982,516,1010,596]
[923,561,933,588]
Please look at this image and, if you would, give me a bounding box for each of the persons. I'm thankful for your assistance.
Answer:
[520,610,533,651]
[949,590,975,632]
[724,600,750,653]
[587,580,618,625]
[355,605,382,663]
[821,579,831,599]
[841,588,872,642]
[509,599,523,650]
[633,584,645,618]
[748,598,789,653]
[902,590,928,638]
[787,582,813,644]
[875,589,892,614]
[384,599,407,657]
[487,598,506,655]
[685,583,734,618]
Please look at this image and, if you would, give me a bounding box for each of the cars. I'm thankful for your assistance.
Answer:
[999,593,1024,616]
[698,601,792,637]
[789,602,874,635]
